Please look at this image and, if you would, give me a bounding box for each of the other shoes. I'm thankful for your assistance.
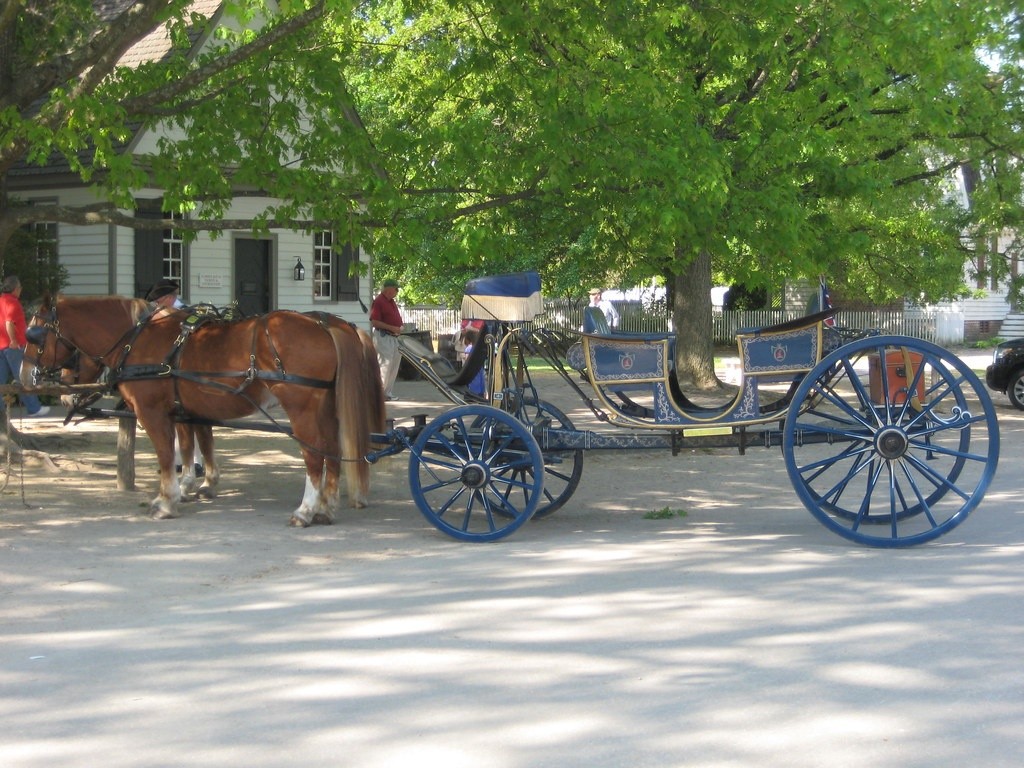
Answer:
[195,463,202,477]
[28,406,50,416]
[174,464,182,473]
[385,395,399,401]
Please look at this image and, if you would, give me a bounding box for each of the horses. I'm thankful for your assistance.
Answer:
[19,281,387,527]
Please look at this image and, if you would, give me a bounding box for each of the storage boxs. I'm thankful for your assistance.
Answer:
[869,349,926,404]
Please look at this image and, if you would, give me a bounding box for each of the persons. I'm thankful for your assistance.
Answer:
[369,279,404,399]
[150,280,203,476]
[0,274,51,417]
[463,330,487,398]
[587,286,619,332]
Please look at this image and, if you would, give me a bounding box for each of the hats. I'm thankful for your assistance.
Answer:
[145,278,179,302]
[384,279,400,287]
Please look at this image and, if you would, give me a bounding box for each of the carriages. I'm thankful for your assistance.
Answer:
[18,270,1001,548]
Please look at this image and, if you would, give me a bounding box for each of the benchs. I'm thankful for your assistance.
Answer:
[735,293,818,373]
[584,307,677,380]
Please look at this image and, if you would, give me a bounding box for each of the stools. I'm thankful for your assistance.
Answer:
[461,271,543,321]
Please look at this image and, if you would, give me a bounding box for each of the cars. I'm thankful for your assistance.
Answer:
[986,337,1024,410]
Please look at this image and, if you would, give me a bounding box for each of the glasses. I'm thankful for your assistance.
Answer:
[392,287,399,292]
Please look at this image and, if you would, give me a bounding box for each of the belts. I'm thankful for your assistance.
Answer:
[376,328,399,338]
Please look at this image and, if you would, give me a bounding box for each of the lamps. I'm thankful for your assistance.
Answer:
[294,256,305,280]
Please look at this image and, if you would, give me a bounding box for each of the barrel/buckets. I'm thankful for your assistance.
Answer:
[399,329,433,381]
[437,334,462,371]
